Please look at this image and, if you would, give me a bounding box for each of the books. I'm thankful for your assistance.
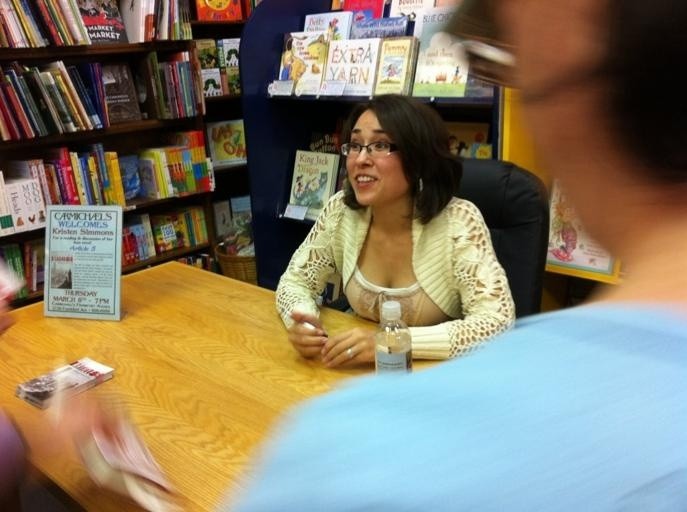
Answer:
[2,0,252,300]
[279,0,494,98]
[291,131,350,222]
[15,356,116,411]
[449,122,492,159]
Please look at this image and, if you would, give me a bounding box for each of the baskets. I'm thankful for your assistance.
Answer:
[214,238,257,286]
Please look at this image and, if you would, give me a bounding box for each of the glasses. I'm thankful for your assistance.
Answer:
[340,140,396,159]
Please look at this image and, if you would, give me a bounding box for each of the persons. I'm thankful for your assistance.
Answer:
[275,93,516,369]
[228,0,687,511]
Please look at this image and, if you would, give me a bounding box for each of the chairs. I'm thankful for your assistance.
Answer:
[457,156,551,315]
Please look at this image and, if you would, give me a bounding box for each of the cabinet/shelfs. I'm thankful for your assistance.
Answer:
[1,0,505,290]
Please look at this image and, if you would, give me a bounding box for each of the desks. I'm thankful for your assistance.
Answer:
[1,262,452,512]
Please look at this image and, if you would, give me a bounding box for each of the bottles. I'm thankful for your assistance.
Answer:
[373,301,413,373]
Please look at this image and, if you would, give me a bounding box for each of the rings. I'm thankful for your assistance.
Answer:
[345,347,355,357]
